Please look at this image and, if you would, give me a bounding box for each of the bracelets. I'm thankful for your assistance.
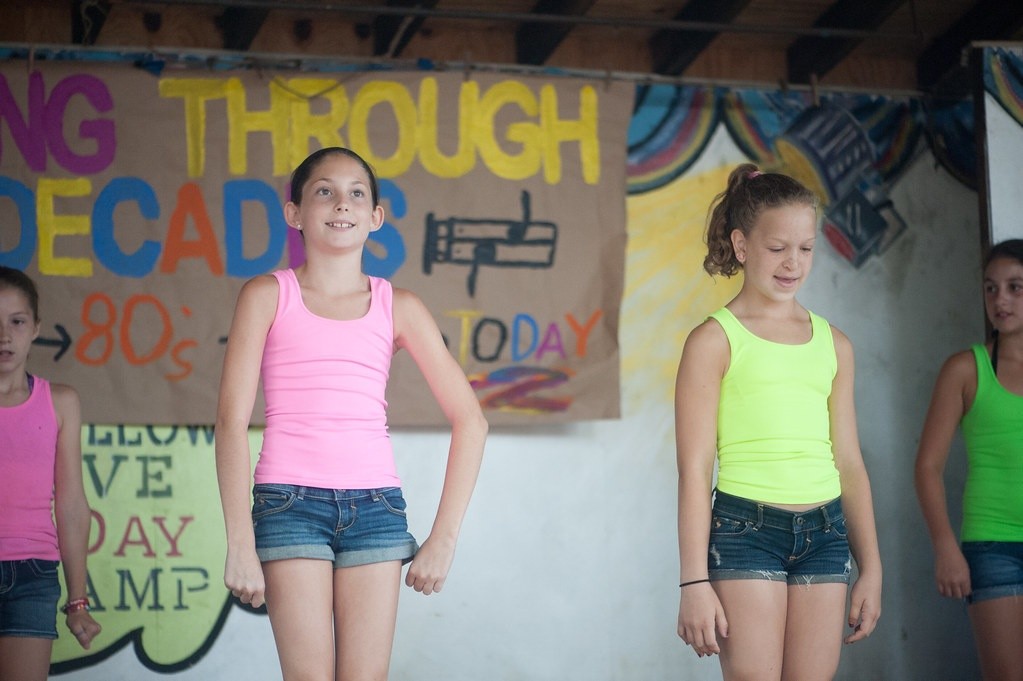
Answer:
[679,579,710,587]
[61,597,90,614]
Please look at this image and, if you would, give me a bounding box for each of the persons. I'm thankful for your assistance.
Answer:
[214,147,490,681]
[674,163,882,681]
[914,238,1023,681]
[0,266,101,681]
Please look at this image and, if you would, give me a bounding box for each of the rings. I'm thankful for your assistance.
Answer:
[76,630,84,637]
